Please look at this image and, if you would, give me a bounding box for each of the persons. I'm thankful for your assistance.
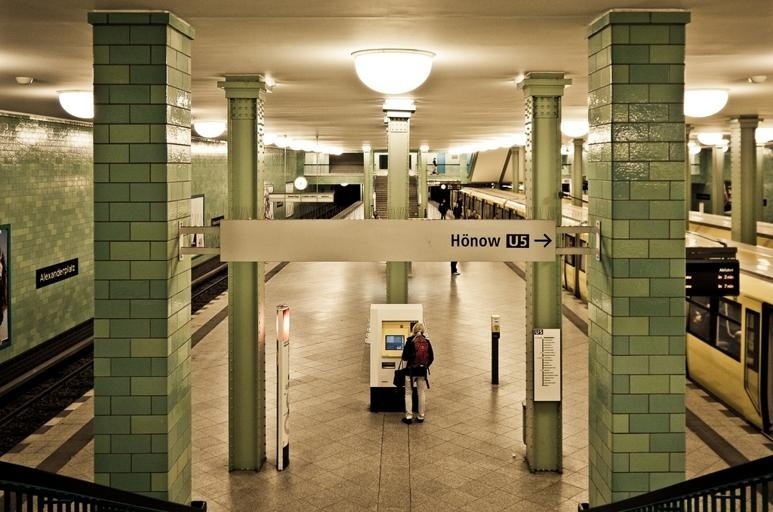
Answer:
[437,198,479,219]
[493,212,502,219]
[451,261,465,274]
[402,322,433,424]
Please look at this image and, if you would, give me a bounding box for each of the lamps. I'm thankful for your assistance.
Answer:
[684,88,729,118]
[562,144,575,155]
[56,90,95,119]
[696,132,724,146]
[261,131,372,155]
[194,120,229,140]
[755,128,772,142]
[418,133,526,155]
[685,139,731,156]
[561,118,588,138]
[350,48,434,98]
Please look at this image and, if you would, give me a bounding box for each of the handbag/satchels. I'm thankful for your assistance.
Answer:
[394,360,405,386]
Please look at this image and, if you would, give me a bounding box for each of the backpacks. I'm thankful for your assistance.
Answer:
[410,332,430,370]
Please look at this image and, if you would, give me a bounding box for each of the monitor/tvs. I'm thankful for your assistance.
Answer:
[385,335,406,351]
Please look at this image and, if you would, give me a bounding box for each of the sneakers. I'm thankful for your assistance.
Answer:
[415,417,423,422]
[403,416,411,424]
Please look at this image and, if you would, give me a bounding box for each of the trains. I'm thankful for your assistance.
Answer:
[448,184,772,439]
